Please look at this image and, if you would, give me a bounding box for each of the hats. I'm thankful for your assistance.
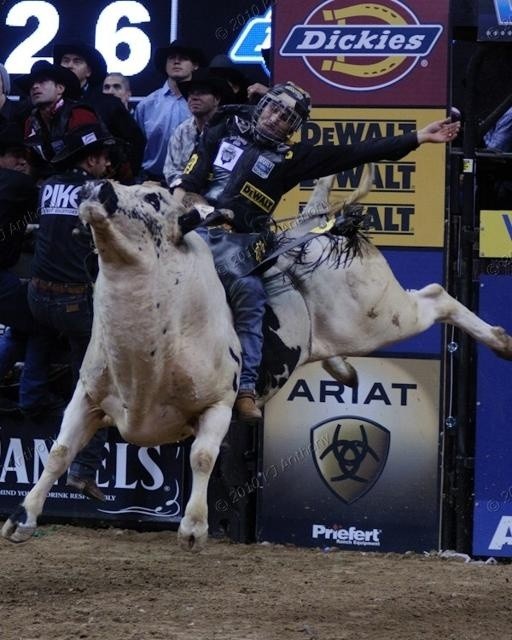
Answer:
[156,40,233,103]
[14,41,107,99]
[51,124,120,163]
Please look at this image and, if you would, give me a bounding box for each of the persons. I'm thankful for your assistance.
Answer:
[169,81,461,419]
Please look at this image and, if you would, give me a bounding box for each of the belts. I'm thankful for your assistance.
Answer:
[31,277,86,295]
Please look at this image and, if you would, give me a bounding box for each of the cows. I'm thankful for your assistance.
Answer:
[0,164,511,555]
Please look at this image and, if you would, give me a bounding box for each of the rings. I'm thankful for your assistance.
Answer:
[448,132,452,137]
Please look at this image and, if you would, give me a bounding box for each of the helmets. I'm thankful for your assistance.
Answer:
[251,80,311,145]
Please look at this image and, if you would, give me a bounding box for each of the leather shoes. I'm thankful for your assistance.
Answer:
[66,475,106,502]
[235,394,262,419]
